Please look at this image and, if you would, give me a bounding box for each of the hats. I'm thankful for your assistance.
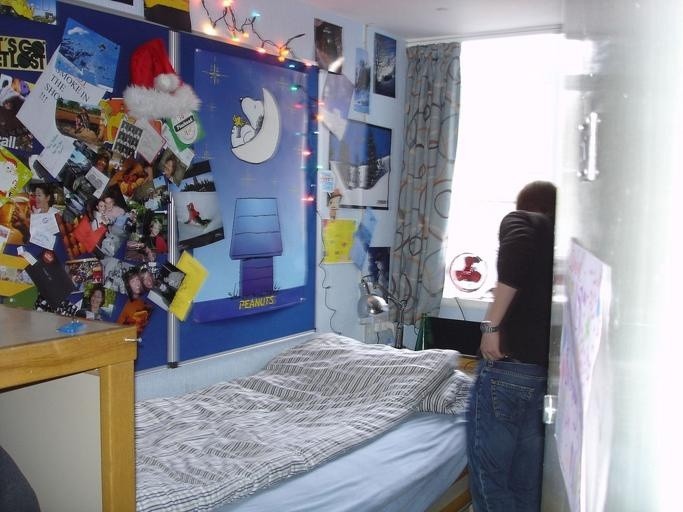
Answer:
[122,39,203,122]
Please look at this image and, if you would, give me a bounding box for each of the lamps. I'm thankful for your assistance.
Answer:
[358,275,407,349]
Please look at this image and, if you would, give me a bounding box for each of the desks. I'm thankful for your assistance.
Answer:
[0,302,138,512]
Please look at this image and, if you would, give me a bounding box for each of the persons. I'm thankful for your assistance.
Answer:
[72,149,212,333]
[12,185,62,245]
[74,106,90,130]
[464,179,557,511]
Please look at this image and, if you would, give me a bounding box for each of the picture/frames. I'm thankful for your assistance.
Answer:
[575,112,600,182]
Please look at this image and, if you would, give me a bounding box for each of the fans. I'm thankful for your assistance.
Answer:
[449,252,489,292]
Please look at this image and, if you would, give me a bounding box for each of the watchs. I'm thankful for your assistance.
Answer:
[479,322,500,334]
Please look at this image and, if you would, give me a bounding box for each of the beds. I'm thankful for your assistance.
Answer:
[134,333,474,512]
